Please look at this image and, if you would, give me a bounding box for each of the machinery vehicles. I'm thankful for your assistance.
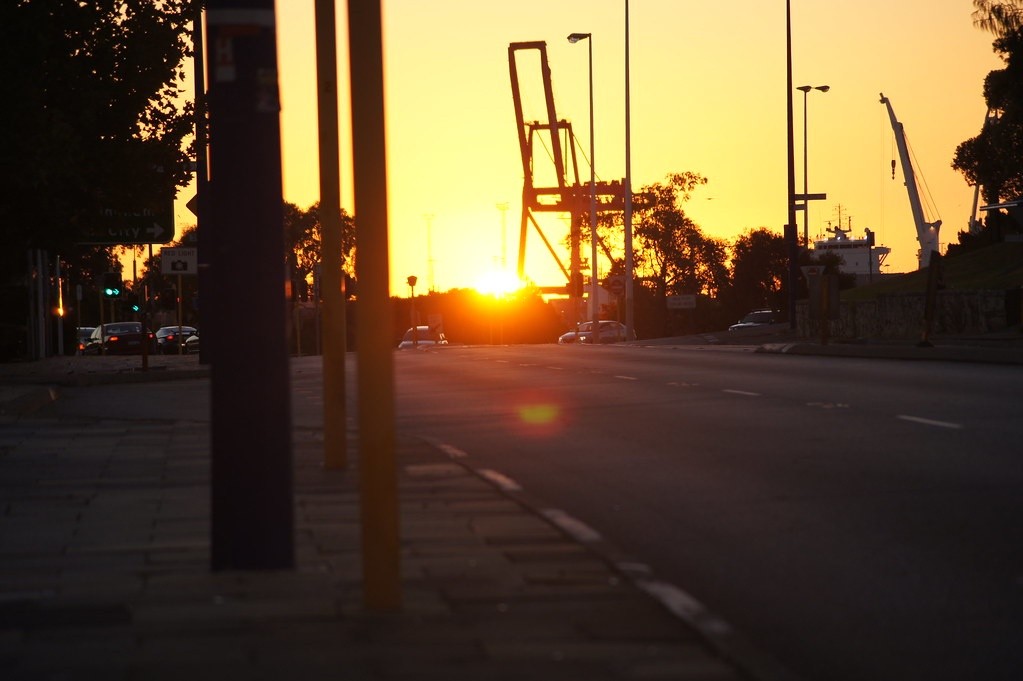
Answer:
[877,91,944,273]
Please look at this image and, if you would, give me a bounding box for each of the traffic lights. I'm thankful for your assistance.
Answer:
[129,294,138,314]
[103,274,122,298]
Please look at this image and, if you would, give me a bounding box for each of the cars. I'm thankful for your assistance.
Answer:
[558,320,638,345]
[729,308,780,333]
[398,326,449,348]
[73,322,199,356]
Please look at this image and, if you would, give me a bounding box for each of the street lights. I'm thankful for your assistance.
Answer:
[797,84,830,248]
[568,32,594,344]
[406,276,418,346]
